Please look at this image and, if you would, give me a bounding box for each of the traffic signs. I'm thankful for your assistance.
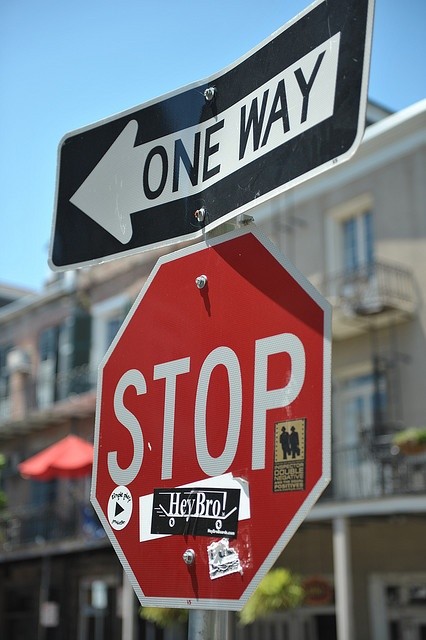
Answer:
[46,1,375,273]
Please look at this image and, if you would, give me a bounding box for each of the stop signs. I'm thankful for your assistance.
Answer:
[91,226,331,610]
